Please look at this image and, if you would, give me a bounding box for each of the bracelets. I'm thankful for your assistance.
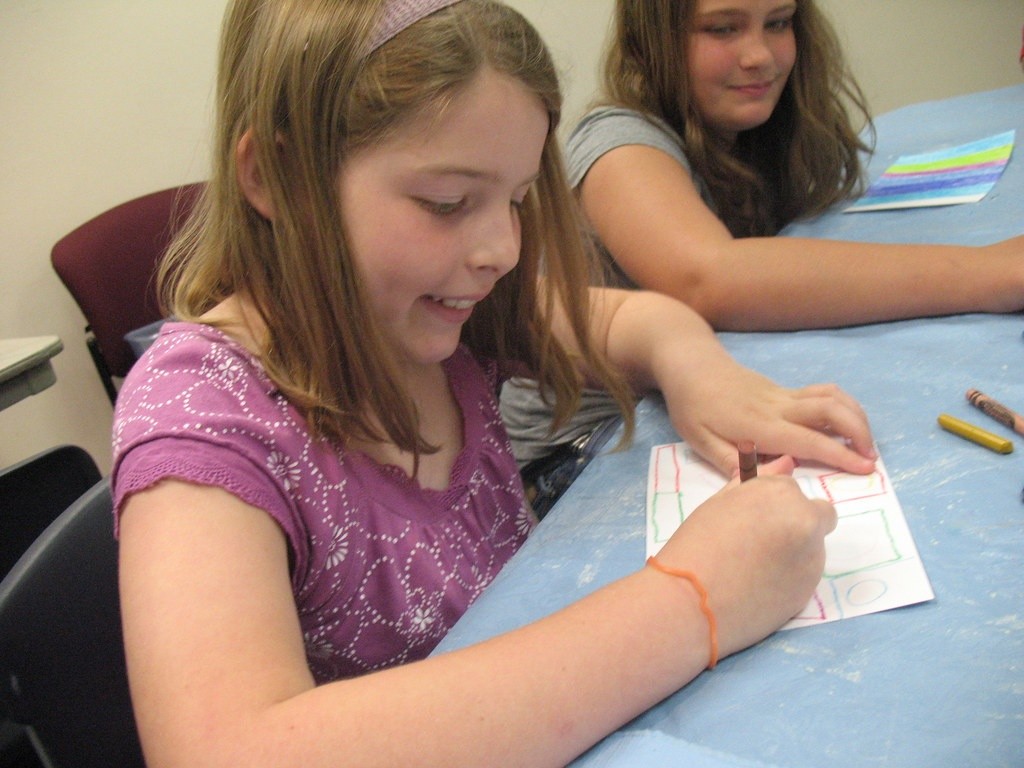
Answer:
[644,556,718,673]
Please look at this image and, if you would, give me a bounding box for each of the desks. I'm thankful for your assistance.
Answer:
[0,336,64,413]
[423,82,1024,768]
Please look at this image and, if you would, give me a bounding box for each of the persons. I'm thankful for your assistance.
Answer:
[559,1,1023,333]
[113,0,880,768]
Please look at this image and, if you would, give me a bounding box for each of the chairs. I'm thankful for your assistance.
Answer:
[47,180,207,407]
[0,442,145,768]
